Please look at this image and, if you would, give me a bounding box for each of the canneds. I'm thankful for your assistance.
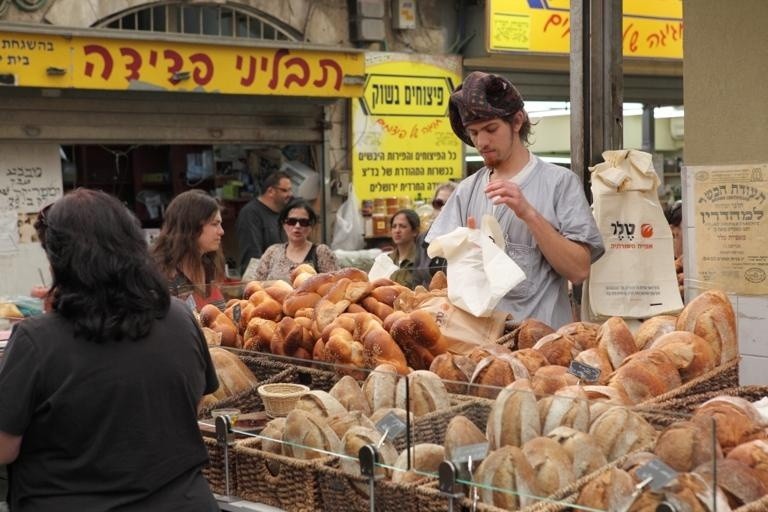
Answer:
[386,197,396,214]
[361,200,373,215]
[373,215,385,235]
[398,197,409,212]
[374,198,385,213]
[385,216,393,236]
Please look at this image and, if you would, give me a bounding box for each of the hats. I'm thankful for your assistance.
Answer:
[448,71,524,146]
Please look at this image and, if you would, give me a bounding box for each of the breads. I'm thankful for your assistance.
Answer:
[577,395,768,512]
[195,346,257,411]
[423,289,739,410]
[187,263,501,373]
[242,364,662,512]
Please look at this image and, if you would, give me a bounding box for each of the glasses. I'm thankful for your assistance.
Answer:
[430,199,444,207]
[275,187,292,191]
[284,216,312,229]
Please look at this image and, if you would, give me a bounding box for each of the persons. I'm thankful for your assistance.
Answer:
[658,198,684,302]
[422,70,605,331]
[253,197,338,283]
[0,185,222,512]
[366,209,420,283]
[234,170,293,273]
[412,183,459,291]
[144,189,243,314]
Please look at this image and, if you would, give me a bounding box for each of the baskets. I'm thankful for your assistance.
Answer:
[198,320,767,512]
